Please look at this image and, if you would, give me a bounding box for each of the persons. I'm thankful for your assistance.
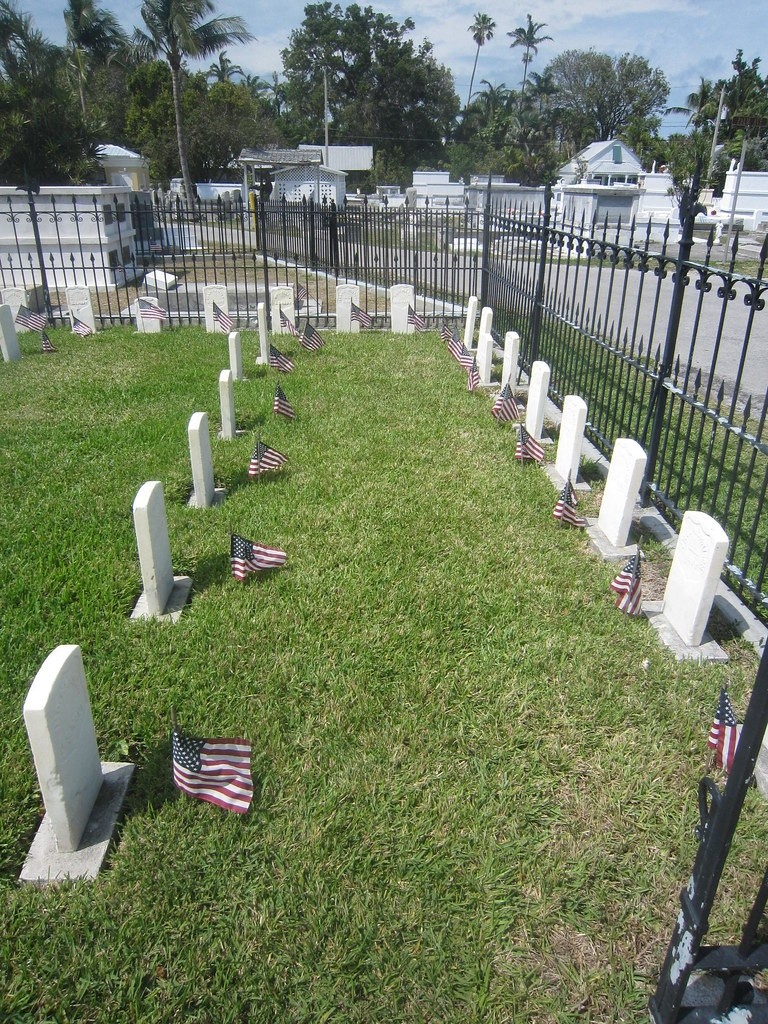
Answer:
[713,220,724,243]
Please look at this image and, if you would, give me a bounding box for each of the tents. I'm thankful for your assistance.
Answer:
[238,147,324,216]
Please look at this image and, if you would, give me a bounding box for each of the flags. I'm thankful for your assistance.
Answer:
[14,305,47,333]
[117,262,129,274]
[273,384,298,420]
[280,309,300,337]
[708,685,741,777]
[138,298,167,319]
[297,282,309,302]
[551,480,590,528]
[491,382,520,423]
[172,732,254,814]
[230,532,287,581]
[248,439,290,477]
[213,302,234,335]
[351,303,372,330]
[610,547,642,617]
[440,323,479,392]
[41,329,59,352]
[299,322,325,352]
[407,304,425,334]
[72,316,92,338]
[514,424,545,463]
[150,239,165,253]
[270,344,295,373]
